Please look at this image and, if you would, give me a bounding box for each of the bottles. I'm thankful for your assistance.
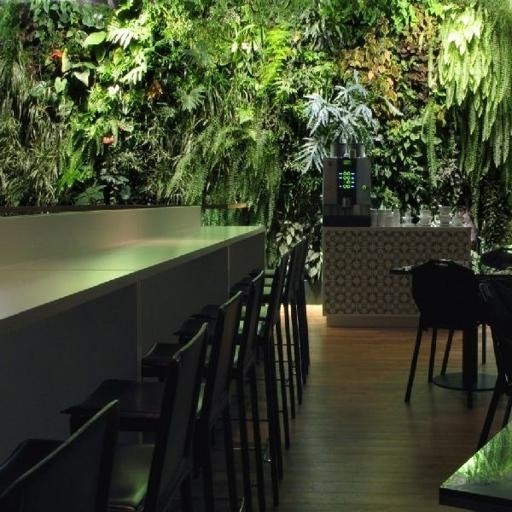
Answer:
[329,142,364,157]
[371,208,401,227]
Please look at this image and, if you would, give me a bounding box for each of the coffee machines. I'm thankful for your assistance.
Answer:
[322,157,371,227]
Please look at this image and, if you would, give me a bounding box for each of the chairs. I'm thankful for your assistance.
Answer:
[479,282,512,449]
[1,311,210,511]
[405,260,475,410]
[61,290,245,510]
[1,379,119,511]
[173,239,310,481]
[139,270,268,511]
[479,249,512,365]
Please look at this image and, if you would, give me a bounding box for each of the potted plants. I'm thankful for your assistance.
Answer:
[295,69,404,157]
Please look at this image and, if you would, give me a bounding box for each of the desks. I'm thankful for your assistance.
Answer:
[390,265,512,391]
[439,422,512,512]
